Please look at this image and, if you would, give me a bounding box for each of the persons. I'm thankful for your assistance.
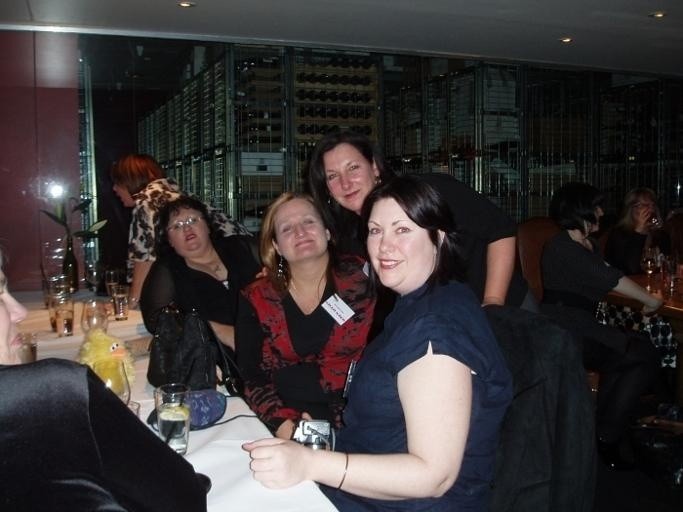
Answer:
[594,186,683,420]
[309,132,519,308]
[138,197,268,380]
[538,181,664,451]
[0,270,211,512]
[241,173,512,512]
[234,190,378,439]
[111,154,254,309]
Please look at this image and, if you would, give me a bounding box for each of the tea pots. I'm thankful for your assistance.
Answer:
[46,276,74,333]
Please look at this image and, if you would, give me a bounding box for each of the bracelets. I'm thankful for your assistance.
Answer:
[337,453,350,490]
[127,295,139,303]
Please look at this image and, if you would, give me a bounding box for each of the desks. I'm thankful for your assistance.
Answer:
[6,289,340,512]
[611,273,681,317]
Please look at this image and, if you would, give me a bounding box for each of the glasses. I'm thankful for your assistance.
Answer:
[166,216,204,232]
[631,202,656,209]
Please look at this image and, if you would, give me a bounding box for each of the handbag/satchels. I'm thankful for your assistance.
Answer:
[145,303,246,399]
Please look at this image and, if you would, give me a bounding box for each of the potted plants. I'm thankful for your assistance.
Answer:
[39,177,94,292]
[75,216,110,288]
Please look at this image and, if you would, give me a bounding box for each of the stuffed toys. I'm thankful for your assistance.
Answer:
[76,329,136,395]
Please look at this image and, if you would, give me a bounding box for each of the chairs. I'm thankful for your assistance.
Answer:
[513,213,609,398]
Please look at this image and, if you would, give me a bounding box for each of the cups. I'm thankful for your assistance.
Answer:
[642,247,659,293]
[92,358,131,406]
[110,283,130,321]
[55,296,77,336]
[80,298,109,333]
[103,268,117,289]
[154,383,191,455]
[87,265,100,289]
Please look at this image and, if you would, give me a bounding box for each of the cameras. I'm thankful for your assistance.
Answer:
[291,417,331,452]
